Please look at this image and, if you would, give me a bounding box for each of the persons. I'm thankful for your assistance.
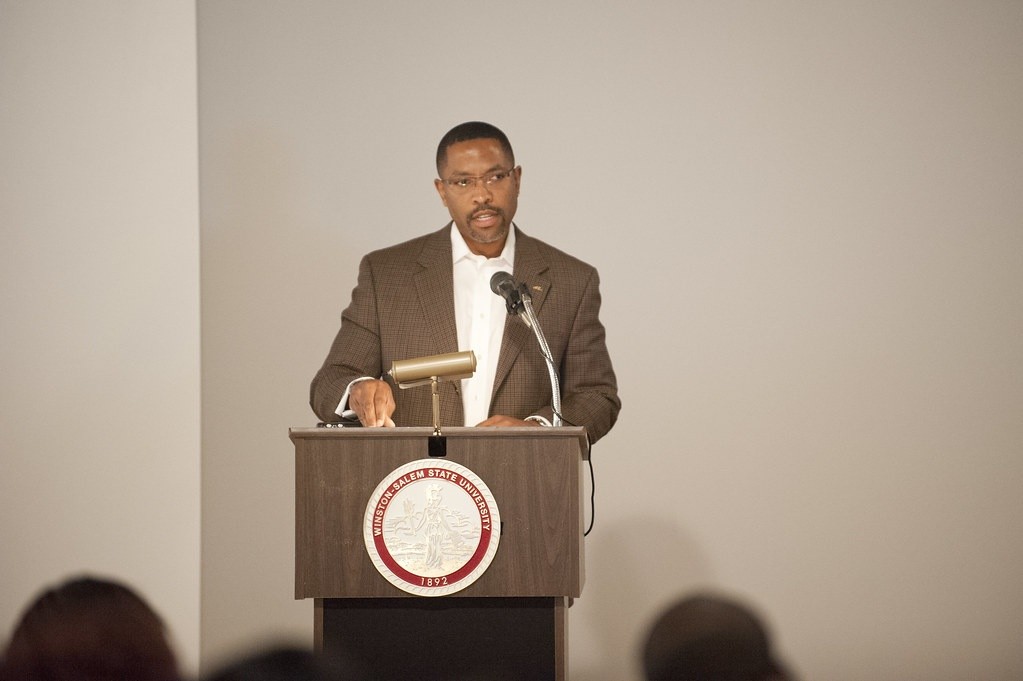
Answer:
[308,121,623,445]
[0,577,799,681]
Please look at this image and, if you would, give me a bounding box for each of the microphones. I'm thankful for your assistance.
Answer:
[489,271,533,331]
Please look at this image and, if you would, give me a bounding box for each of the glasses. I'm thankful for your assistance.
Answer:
[439,166,515,194]
